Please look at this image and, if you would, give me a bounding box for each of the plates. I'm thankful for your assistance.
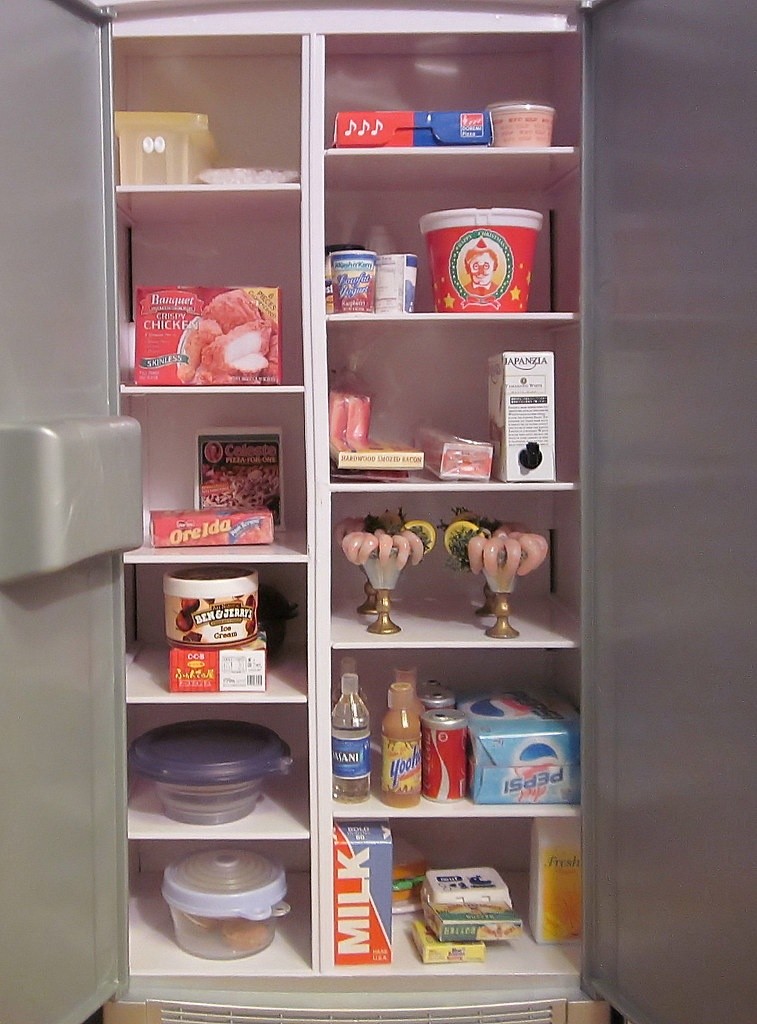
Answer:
[201,168,297,184]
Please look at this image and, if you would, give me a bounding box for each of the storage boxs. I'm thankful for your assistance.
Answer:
[329,350,556,485]
[333,110,494,149]
[149,505,274,546]
[131,283,282,386]
[192,424,285,533]
[169,627,267,692]
[333,818,585,965]
[456,683,579,805]
[115,109,211,184]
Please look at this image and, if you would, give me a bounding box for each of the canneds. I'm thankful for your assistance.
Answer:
[414,679,469,802]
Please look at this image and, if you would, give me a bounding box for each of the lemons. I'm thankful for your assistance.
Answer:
[444,521,484,563]
[401,521,435,557]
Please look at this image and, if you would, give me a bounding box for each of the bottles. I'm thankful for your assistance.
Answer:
[332,658,372,717]
[382,682,422,808]
[331,674,372,804]
[393,667,426,720]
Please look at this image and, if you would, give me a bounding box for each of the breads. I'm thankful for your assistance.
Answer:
[327,388,373,443]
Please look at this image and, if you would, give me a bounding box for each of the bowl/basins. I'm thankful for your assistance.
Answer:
[131,719,295,826]
[162,848,292,959]
